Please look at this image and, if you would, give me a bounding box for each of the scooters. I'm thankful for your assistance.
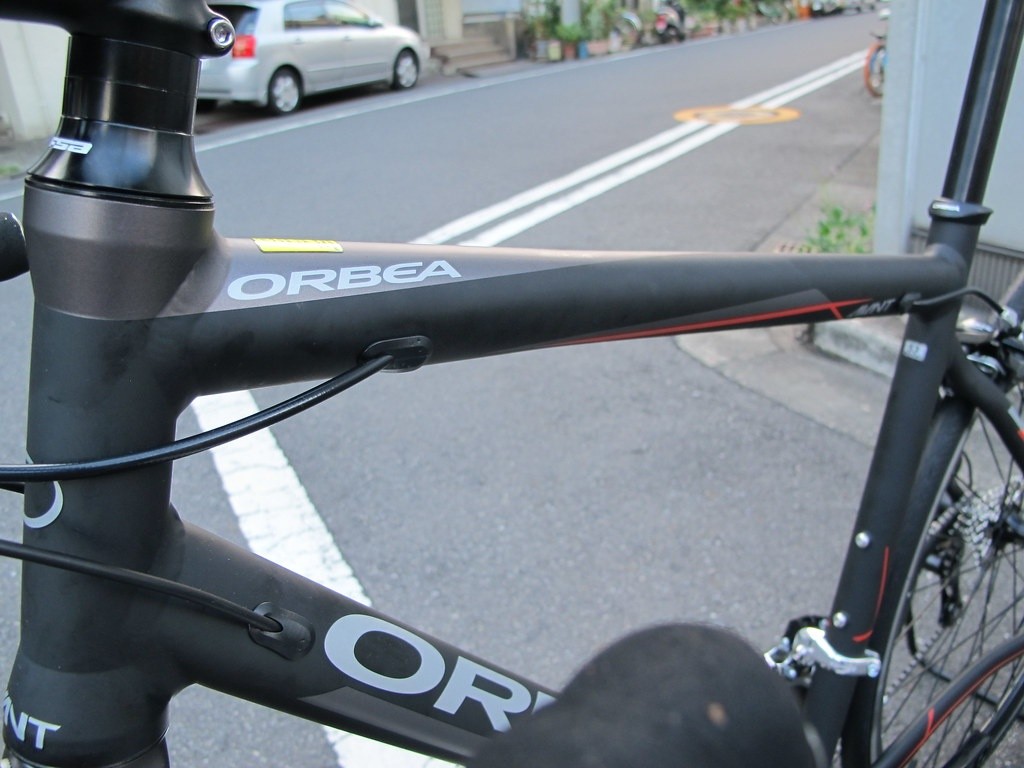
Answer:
[653,0,686,46]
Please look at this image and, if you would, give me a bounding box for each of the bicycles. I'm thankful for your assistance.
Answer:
[0,0,1024,768]
[863,9,890,99]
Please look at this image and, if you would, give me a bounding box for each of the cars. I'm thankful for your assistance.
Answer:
[195,0,424,117]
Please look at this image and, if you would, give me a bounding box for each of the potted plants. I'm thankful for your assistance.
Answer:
[520,0,622,65]
